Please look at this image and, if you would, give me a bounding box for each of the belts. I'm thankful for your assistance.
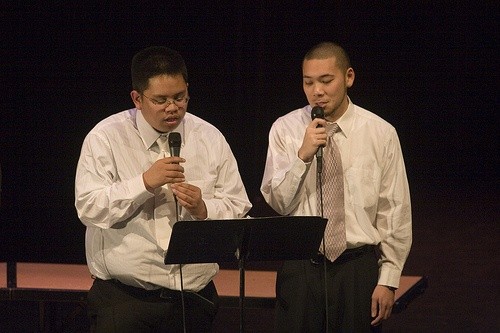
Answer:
[106,279,215,312]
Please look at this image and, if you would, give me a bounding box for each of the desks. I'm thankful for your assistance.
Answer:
[0,262,427,333]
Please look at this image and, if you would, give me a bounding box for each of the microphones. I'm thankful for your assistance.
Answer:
[168,132,182,198]
[311,106,324,174]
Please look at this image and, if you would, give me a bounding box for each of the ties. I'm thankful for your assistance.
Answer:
[317,123,347,262]
[155,136,180,252]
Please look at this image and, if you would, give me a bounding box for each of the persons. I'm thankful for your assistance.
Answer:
[75,47,253,333]
[260,42,413,333]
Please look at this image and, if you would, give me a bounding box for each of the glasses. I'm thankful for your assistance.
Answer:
[140,92,190,107]
